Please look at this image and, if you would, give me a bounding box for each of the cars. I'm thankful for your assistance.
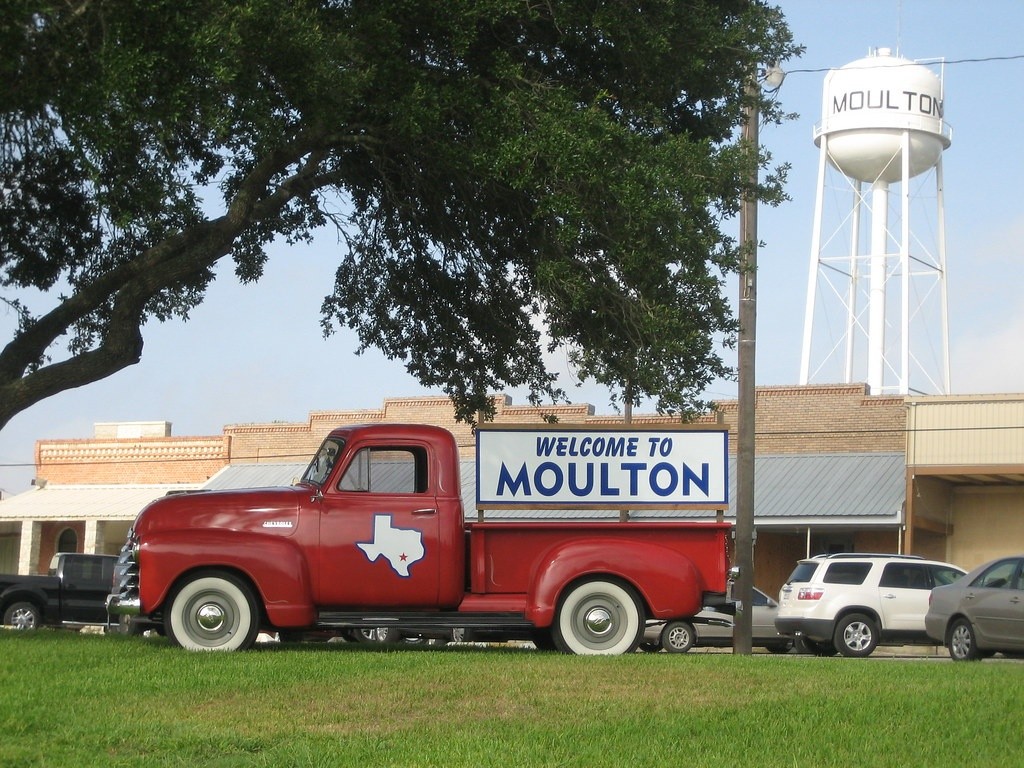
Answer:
[644,581,796,654]
[775,551,971,659]
[924,555,1024,663]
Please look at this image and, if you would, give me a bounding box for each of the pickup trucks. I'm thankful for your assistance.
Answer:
[102,423,735,657]
[0,552,152,635]
